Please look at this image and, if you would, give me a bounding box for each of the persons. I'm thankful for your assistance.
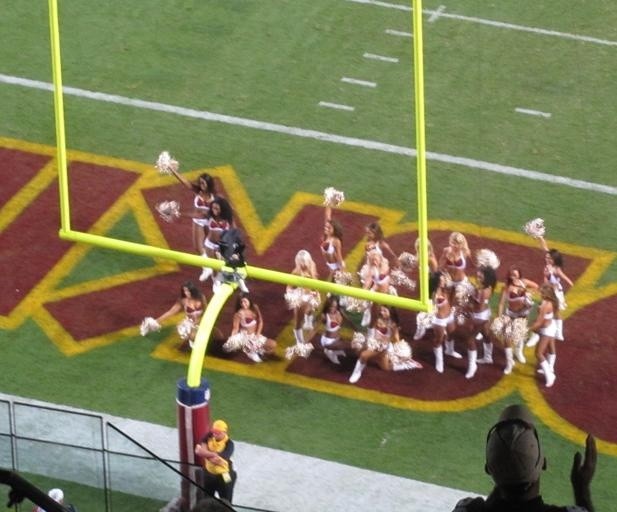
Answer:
[451,405,597,512]
[152,162,278,355]
[32,488,79,512]
[285,203,422,383]
[194,419,237,505]
[410,236,574,388]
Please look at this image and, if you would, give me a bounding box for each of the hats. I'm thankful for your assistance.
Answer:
[211,420,228,434]
[485,405,545,493]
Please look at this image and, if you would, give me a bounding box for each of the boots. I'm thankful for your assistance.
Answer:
[237,279,250,294]
[323,348,346,365]
[293,328,305,345]
[464,330,557,388]
[554,319,564,341]
[413,312,427,341]
[432,344,445,374]
[361,309,371,327]
[212,276,222,293]
[198,251,212,282]
[247,351,263,362]
[443,338,464,359]
[302,312,314,331]
[348,359,367,384]
